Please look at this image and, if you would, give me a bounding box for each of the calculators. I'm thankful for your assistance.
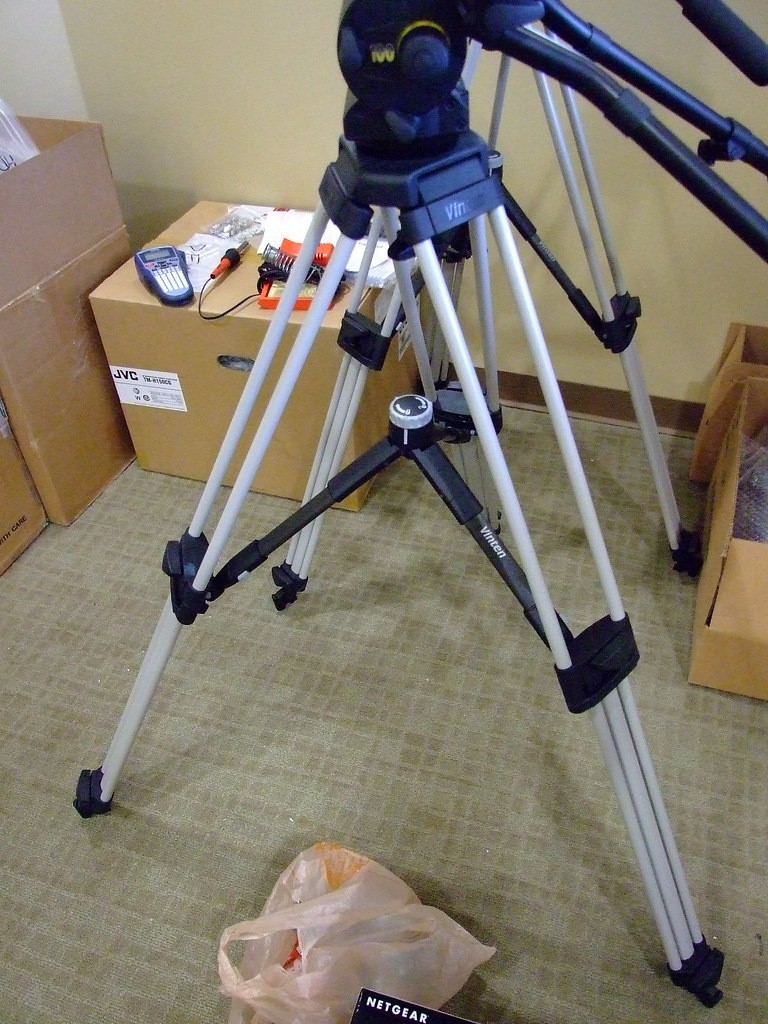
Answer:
[134,245,195,306]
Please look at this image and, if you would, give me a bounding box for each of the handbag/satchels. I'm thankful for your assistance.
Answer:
[217,841,496,1024]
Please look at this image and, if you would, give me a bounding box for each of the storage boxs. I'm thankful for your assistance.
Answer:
[0,114,137,577]
[89,198,415,512]
[688,322,768,702]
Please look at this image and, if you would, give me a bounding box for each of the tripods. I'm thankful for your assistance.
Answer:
[73,0,768,1008]
